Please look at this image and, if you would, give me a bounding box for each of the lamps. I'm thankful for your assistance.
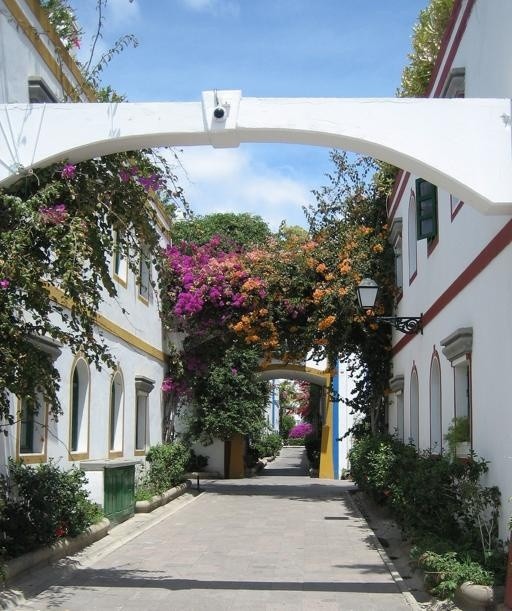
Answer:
[355,271,423,335]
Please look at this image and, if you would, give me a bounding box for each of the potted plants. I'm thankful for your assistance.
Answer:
[442,414,472,459]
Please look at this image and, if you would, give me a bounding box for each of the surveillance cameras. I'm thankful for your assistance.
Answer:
[213,105,226,121]
